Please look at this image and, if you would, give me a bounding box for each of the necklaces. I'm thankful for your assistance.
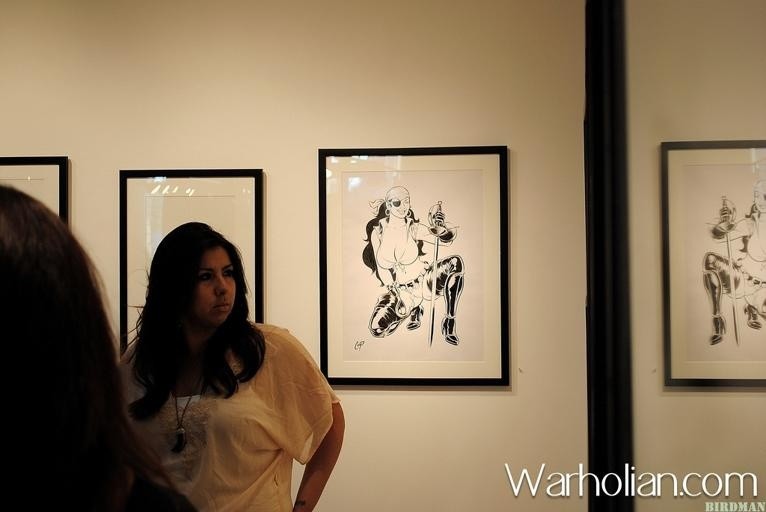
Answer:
[170,360,202,454]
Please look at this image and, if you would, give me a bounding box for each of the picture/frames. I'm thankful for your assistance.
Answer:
[0,147,510,388]
[660,140,766,389]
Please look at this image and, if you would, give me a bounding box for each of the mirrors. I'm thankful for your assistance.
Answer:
[584,0,766,512]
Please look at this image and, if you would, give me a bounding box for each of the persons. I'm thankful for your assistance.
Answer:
[357,184,466,346]
[112,218,347,511]
[0,180,203,510]
[696,177,766,346]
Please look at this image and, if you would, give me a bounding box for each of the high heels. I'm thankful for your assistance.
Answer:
[743,304,762,329]
[406,305,424,331]
[708,313,727,347]
[440,315,459,346]
